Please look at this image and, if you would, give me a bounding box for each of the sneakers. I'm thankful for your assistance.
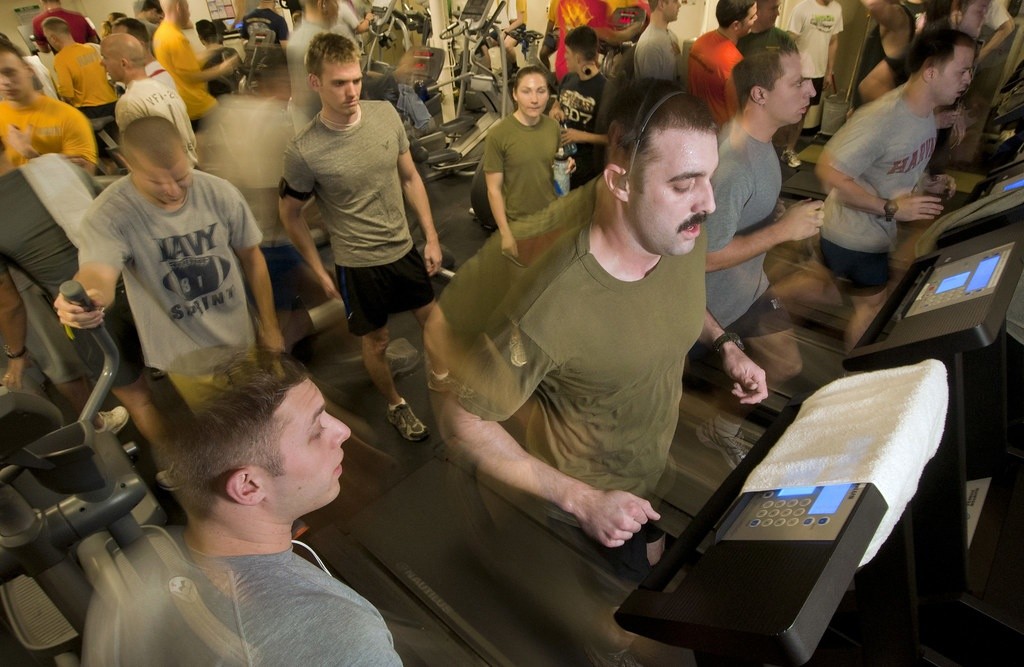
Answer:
[426,371,475,398]
[780,149,801,168]
[385,397,429,442]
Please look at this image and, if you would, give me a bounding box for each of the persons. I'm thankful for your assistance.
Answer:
[0,1,239,178]
[1,115,406,667]
[242,0,578,442]
[422,0,1024,666]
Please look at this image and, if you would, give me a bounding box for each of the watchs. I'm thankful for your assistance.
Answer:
[883,198,899,222]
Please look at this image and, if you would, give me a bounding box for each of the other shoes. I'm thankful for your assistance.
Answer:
[773,200,787,222]
[510,328,527,366]
[581,632,640,667]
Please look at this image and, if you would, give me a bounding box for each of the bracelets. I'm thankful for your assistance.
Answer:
[364,18,372,24]
[3,345,27,358]
[712,332,744,356]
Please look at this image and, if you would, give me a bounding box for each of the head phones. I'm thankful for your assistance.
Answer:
[618,88,689,191]
